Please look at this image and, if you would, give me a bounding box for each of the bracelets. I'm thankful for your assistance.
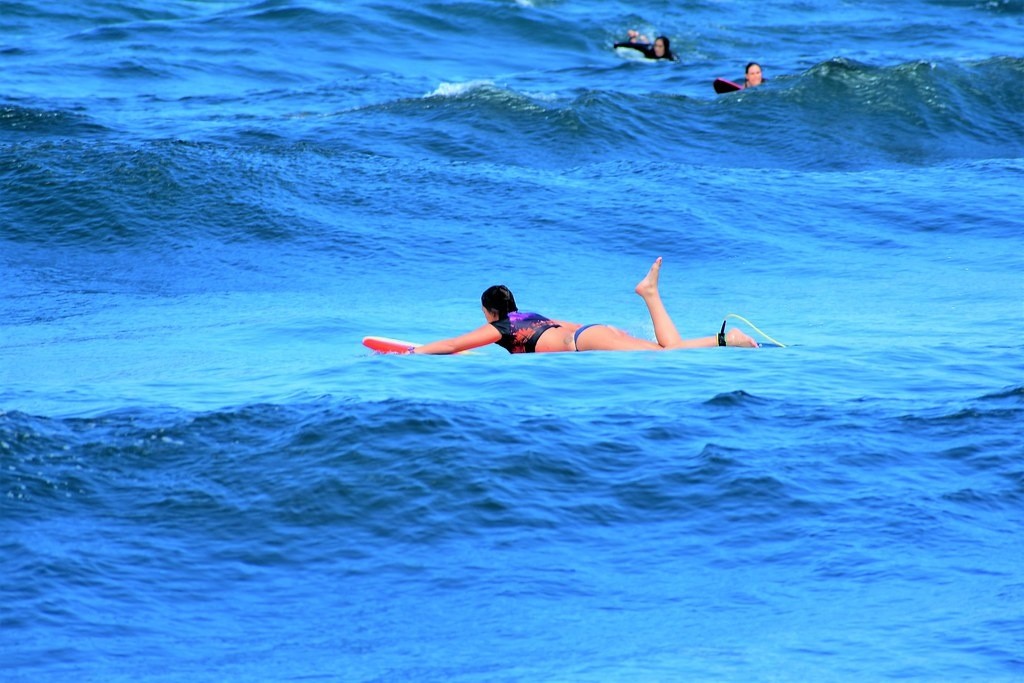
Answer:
[408,346,414,354]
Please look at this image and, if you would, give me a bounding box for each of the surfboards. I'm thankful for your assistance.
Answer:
[713,78,743,95]
[361,335,475,356]
[617,47,657,63]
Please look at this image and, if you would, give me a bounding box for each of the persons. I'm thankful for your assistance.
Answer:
[405,256,759,354]
[743,62,762,88]
[612,30,673,61]
